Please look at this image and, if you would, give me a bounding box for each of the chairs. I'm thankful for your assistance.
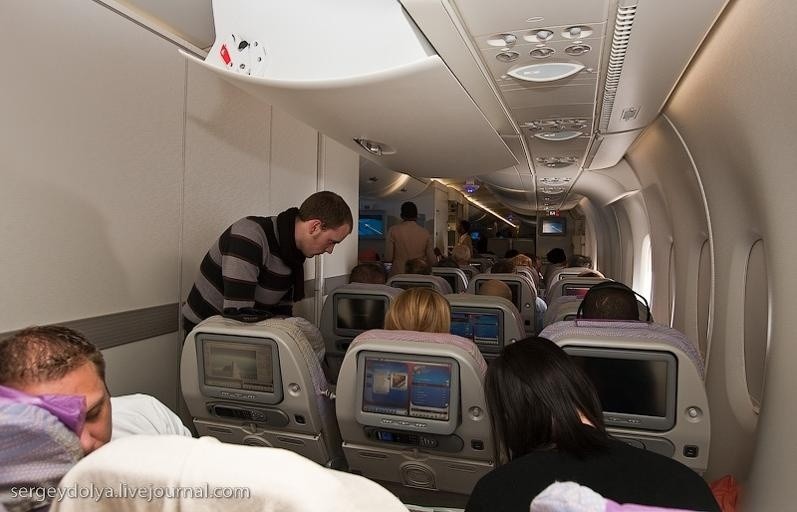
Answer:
[0,253,711,512]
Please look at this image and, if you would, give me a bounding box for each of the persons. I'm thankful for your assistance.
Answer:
[350,200,644,334]
[465,336,720,512]
[0,324,194,459]
[178,190,353,345]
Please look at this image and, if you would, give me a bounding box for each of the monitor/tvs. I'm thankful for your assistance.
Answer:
[541,216,565,235]
[448,305,504,355]
[354,350,460,436]
[470,231,480,240]
[561,347,678,432]
[358,209,388,240]
[562,284,597,297]
[195,333,283,406]
[433,273,458,294]
[475,279,520,310]
[469,263,482,273]
[333,293,390,338]
[391,281,434,291]
